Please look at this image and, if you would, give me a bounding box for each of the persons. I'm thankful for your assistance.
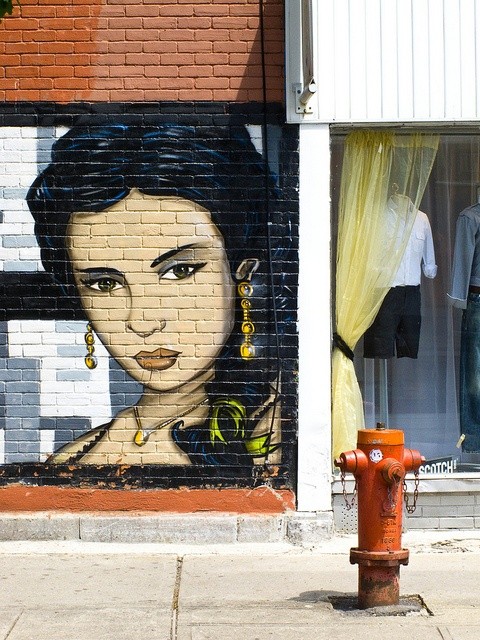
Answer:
[25,124,283,465]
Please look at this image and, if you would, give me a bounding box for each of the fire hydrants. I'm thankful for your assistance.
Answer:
[334,422,426,610]
